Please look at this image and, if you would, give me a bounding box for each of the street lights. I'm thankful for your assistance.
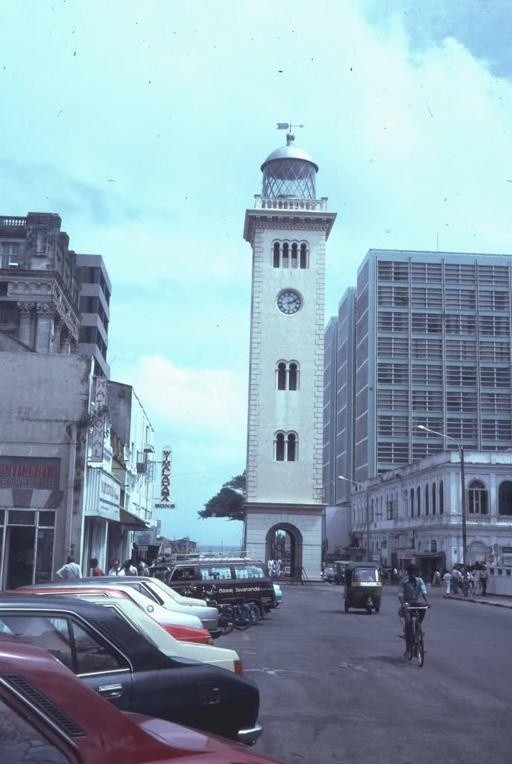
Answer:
[338,476,369,560]
[417,424,467,578]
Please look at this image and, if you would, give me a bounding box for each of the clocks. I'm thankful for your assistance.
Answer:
[277,291,300,315]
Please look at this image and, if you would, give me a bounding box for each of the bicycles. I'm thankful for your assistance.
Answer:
[397,602,429,667]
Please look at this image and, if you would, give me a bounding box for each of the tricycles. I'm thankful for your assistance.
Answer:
[343,562,383,615]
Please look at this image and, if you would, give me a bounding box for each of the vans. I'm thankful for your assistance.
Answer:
[147,557,277,616]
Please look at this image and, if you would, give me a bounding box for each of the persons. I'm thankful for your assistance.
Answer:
[268,556,282,577]
[55,556,152,581]
[430,566,490,597]
[397,566,432,657]
